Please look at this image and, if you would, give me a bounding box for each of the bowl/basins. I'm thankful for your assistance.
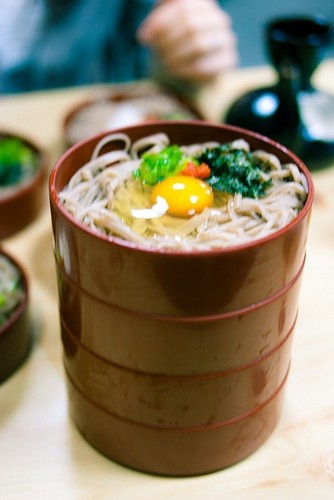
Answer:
[0,131,48,240]
[0,249,30,383]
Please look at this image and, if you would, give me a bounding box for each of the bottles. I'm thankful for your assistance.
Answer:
[224,16,334,172]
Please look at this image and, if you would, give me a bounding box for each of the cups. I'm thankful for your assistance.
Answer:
[49,120,315,477]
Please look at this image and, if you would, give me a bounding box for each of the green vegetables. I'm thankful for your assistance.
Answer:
[133,142,273,194]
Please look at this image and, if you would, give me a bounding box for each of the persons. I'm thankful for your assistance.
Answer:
[1,0,237,96]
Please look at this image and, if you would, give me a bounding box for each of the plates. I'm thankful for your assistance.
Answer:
[64,89,207,146]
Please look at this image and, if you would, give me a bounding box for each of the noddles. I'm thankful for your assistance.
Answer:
[56,132,309,249]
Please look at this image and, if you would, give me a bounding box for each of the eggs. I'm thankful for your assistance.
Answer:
[108,159,233,233]
